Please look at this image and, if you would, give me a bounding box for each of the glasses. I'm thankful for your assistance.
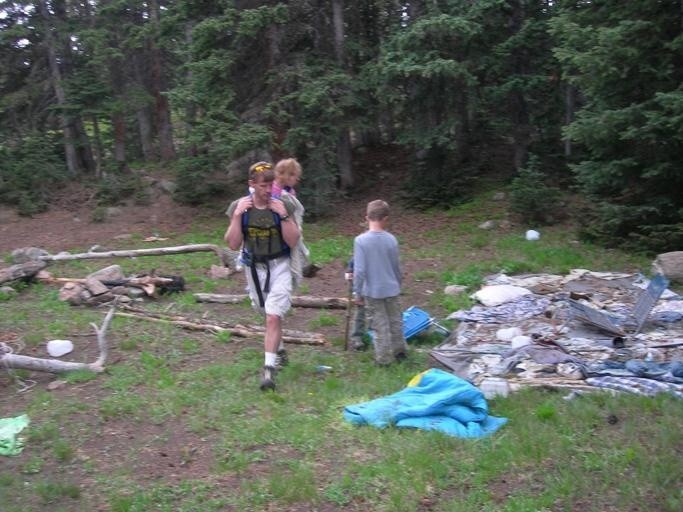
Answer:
[251,164,273,175]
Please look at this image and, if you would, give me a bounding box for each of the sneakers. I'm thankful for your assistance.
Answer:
[276,349,289,367]
[374,352,407,369]
[261,367,275,390]
[352,341,365,351]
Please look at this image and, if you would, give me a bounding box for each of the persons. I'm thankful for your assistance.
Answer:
[345,200,407,369]
[224,158,302,391]
